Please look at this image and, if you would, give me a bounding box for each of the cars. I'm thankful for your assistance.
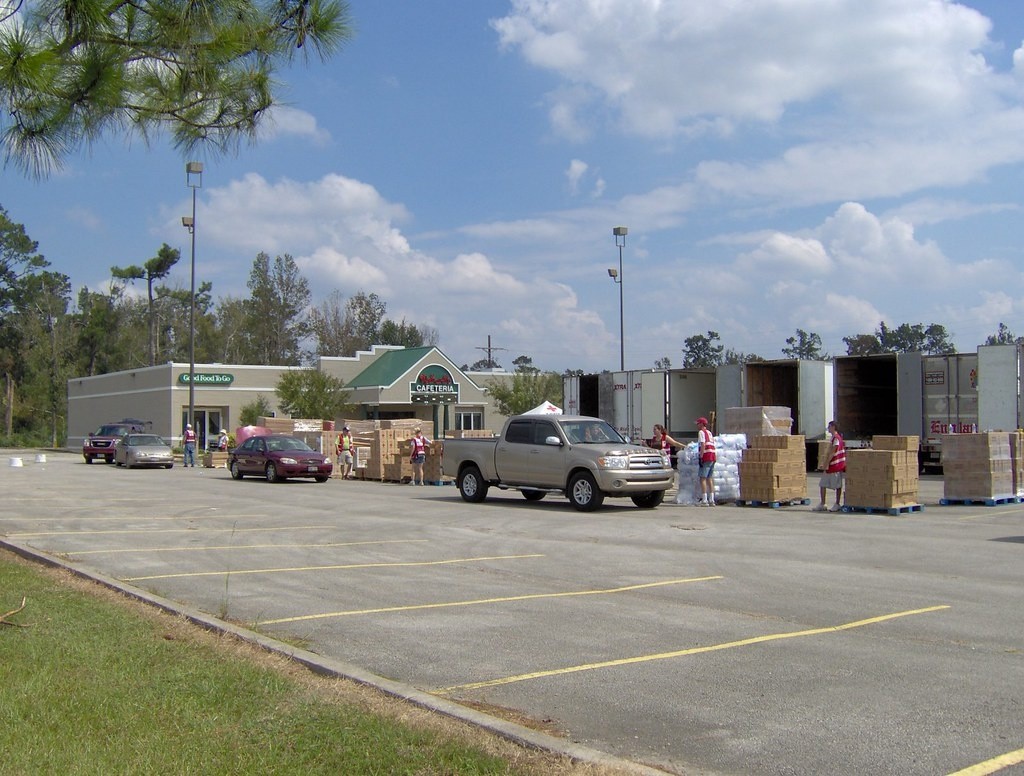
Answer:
[226,434,333,482]
[112,433,174,469]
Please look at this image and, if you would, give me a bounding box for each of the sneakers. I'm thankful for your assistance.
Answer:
[829,503,841,512]
[813,504,828,510]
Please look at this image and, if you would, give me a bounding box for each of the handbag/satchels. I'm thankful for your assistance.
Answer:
[222,442,225,448]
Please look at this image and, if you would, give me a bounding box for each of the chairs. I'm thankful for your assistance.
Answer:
[572,429,596,440]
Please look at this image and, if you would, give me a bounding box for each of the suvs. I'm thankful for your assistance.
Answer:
[83,418,152,464]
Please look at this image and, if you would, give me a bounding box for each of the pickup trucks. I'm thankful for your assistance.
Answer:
[441,414,676,513]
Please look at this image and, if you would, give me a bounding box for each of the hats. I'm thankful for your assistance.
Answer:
[694,418,708,425]
[187,424,191,427]
[220,429,227,435]
[343,427,350,431]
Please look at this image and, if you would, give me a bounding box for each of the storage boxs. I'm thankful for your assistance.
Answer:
[942,432,1024,499]
[257,417,493,481]
[725,404,806,501]
[845,435,919,507]
[818,441,832,470]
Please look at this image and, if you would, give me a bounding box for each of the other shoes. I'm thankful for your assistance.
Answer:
[408,481,415,486]
[418,481,424,485]
[345,474,350,480]
[708,500,715,506]
[695,500,710,507]
[342,476,345,480]
[183,464,188,467]
[191,463,194,467]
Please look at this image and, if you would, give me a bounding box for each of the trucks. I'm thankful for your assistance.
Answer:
[561,345,1024,475]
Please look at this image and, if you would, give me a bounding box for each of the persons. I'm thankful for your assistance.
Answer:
[812,421,847,512]
[408,427,432,486]
[335,427,356,480]
[183,423,197,467]
[641,424,686,468]
[218,429,229,452]
[693,417,716,507]
[580,423,601,442]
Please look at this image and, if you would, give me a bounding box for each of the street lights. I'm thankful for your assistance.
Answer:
[181,161,204,453]
[608,226,629,371]
